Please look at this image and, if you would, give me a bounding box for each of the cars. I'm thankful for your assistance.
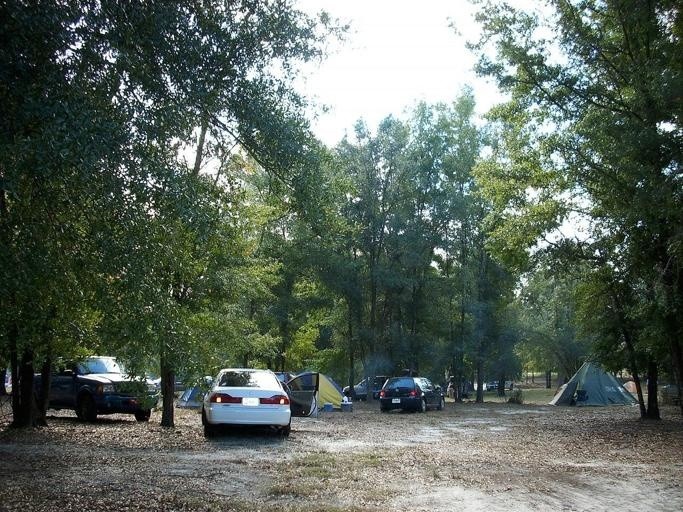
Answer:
[487,377,514,391]
[199,368,319,440]
[443,375,471,398]
[153,375,186,392]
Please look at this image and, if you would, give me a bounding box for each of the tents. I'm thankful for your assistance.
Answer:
[176,376,214,408]
[283,372,345,409]
[548,361,639,407]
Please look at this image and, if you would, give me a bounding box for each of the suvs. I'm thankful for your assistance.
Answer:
[377,375,445,412]
[342,375,386,399]
[33,353,160,425]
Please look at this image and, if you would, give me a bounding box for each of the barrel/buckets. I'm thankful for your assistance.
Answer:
[341,402,352,412]
[324,403,333,411]
